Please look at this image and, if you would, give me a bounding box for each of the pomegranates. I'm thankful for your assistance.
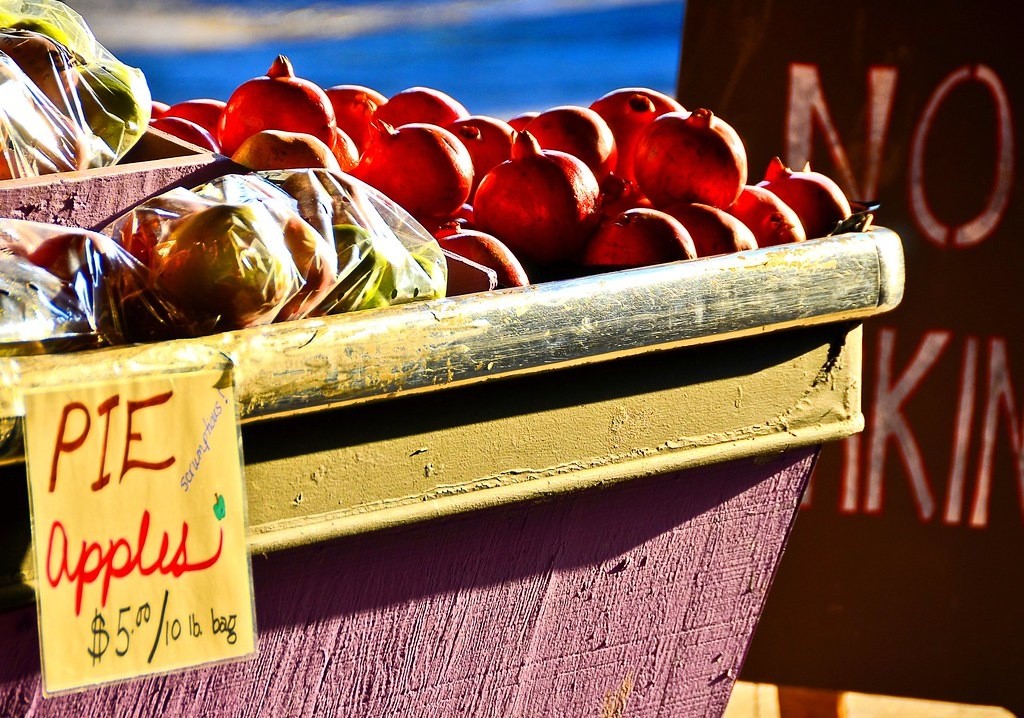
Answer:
[137,54,853,294]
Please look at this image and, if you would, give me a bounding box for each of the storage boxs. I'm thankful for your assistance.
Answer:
[0,157,908,718]
